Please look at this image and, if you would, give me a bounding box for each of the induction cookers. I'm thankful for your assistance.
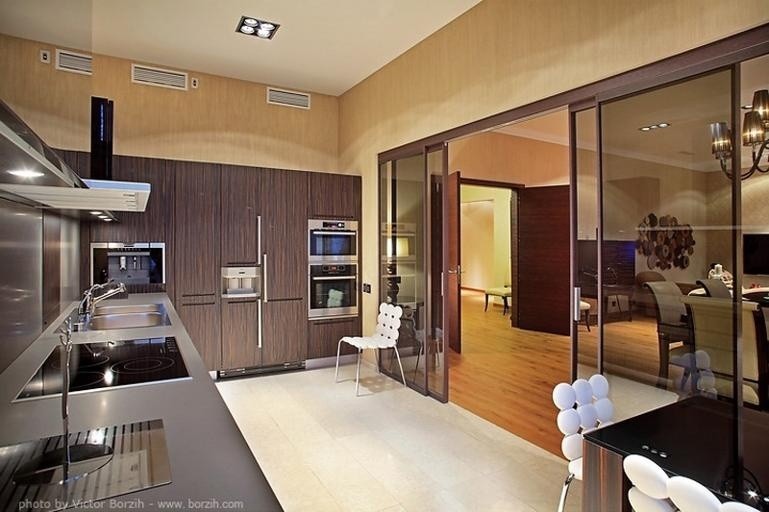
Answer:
[10,335,192,409]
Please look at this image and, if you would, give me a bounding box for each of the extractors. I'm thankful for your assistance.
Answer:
[0,177,153,214]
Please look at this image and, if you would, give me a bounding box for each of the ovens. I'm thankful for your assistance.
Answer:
[307,218,360,321]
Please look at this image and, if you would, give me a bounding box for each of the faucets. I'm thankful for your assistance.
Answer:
[82,278,127,313]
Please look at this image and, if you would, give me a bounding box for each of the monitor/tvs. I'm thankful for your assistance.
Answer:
[743,233,769,276]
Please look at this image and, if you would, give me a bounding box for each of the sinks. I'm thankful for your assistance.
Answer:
[72,302,165,318]
[53,313,172,334]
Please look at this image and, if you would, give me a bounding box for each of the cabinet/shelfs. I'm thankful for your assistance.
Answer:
[56,146,362,373]
[581,288,633,324]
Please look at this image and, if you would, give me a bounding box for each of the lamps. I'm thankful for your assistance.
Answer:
[234,15,280,41]
[709,88,769,181]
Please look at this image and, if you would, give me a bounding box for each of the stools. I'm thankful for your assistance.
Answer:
[580,300,591,332]
[485,288,512,315]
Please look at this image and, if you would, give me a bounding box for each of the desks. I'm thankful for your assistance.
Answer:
[580,393,769,512]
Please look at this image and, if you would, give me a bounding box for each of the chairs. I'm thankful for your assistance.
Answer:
[332,302,408,397]
[678,294,769,412]
[695,279,731,298]
[621,454,761,512]
[552,374,616,512]
[642,279,690,390]
[636,271,695,317]
